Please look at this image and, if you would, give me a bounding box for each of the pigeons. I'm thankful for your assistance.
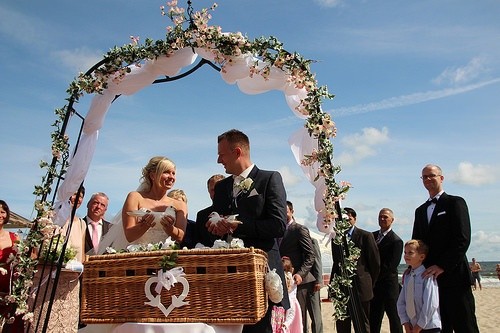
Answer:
[209,212,243,226]
[127,206,176,222]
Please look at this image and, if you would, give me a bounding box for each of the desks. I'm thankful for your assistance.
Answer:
[78,322,243,333]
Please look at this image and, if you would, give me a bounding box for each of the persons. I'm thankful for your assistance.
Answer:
[415,165,480,332]
[212,128,289,332]
[0,176,403,333]
[471,256,500,291]
[398,242,441,332]
[122,156,186,249]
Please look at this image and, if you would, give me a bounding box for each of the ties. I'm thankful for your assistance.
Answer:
[406,272,416,319]
[375,233,384,243]
[90,221,99,255]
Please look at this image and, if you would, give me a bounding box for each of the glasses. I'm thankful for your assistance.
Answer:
[420,174,441,179]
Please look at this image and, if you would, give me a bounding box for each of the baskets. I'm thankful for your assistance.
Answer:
[80,246,268,323]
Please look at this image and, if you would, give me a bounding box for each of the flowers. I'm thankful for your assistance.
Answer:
[3,0,364,333]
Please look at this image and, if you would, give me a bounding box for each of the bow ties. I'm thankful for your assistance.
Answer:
[426,197,437,207]
